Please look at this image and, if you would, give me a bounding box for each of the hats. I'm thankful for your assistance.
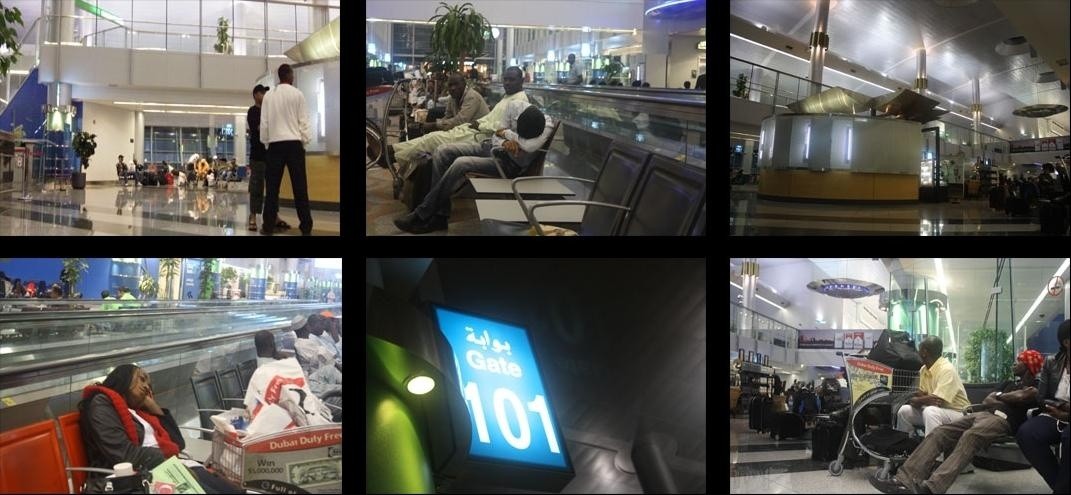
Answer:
[253,84,269,92]
[219,158,227,161]
[319,311,337,320]
[1017,349,1045,379]
[291,314,308,330]
[1057,319,1071,340]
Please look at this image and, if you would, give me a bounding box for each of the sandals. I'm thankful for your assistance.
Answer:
[274,221,291,229]
[249,215,257,230]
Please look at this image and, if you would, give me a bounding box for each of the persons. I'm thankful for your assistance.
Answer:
[367,67,529,169]
[255,311,342,425]
[392,99,553,234]
[184,154,238,182]
[259,63,313,234]
[247,84,291,231]
[562,53,583,86]
[894,335,975,474]
[869,350,1044,494]
[10,277,50,299]
[116,155,143,187]
[418,73,490,134]
[469,63,477,79]
[92,291,121,335]
[1016,319,1071,495]
[78,364,254,495]
[119,286,143,311]
[154,160,169,185]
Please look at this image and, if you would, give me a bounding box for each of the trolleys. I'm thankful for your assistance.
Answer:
[209,401,342,495]
[829,352,918,476]
[366,79,411,199]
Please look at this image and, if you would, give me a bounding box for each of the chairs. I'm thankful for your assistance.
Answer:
[0,420,142,495]
[207,165,246,187]
[448,141,650,237]
[215,366,246,413]
[189,370,229,443]
[118,162,163,183]
[528,152,707,236]
[236,358,257,392]
[894,381,1062,467]
[57,411,191,493]
[453,112,561,198]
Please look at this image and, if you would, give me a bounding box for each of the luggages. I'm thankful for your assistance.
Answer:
[749,396,774,433]
[793,387,819,425]
[767,411,805,440]
[812,421,844,462]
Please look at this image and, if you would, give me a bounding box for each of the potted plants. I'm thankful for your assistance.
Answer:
[70,128,97,189]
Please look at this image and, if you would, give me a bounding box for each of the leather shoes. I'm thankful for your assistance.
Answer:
[367,146,393,169]
[869,476,908,494]
[259,229,273,236]
[892,485,932,495]
[393,211,425,230]
[302,230,310,236]
[410,215,448,234]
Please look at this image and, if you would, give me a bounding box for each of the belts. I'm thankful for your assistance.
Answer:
[504,154,522,174]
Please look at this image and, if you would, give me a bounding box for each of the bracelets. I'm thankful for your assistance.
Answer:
[501,129,506,138]
